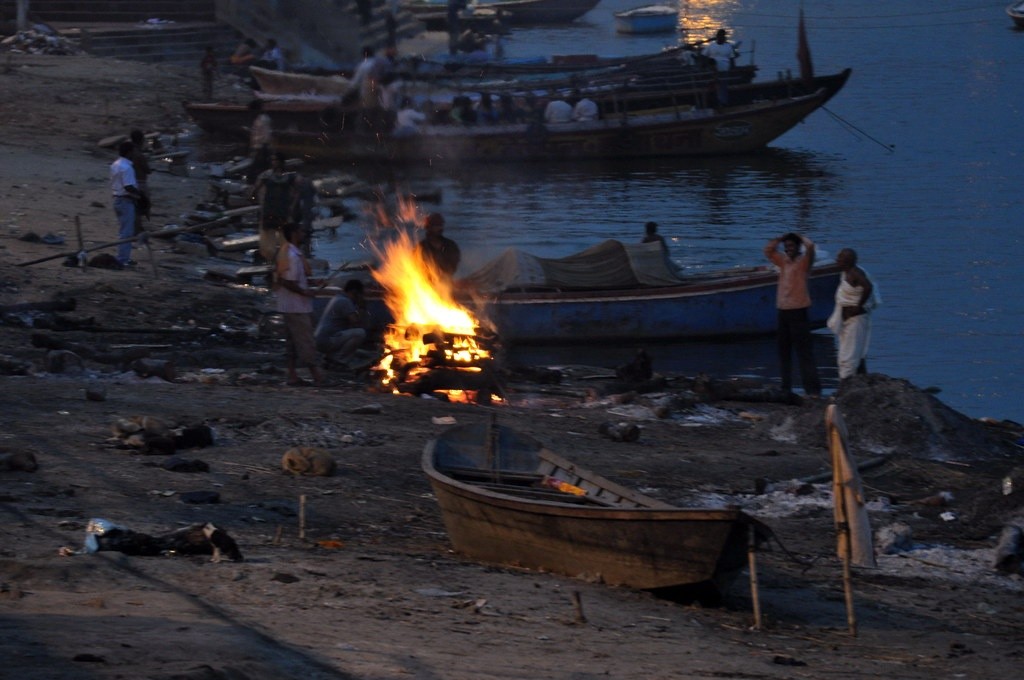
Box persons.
[231,38,285,90]
[274,224,327,384]
[244,103,274,182]
[200,47,217,97]
[343,44,598,130]
[677,28,737,73]
[109,141,140,265]
[131,130,154,234]
[249,158,316,265]
[417,212,460,276]
[641,221,668,249]
[827,247,880,388]
[314,279,367,359]
[765,231,822,399]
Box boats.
[615,5,679,34]
[314,264,859,342]
[1005,0,1024,30]
[420,422,771,590]
[408,0,601,22]
[184,44,853,165]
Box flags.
[795,19,813,80]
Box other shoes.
[127,258,137,265]
[810,391,820,399]
[284,377,310,386]
[121,260,133,269]
[322,354,335,362]
[311,377,340,387]
